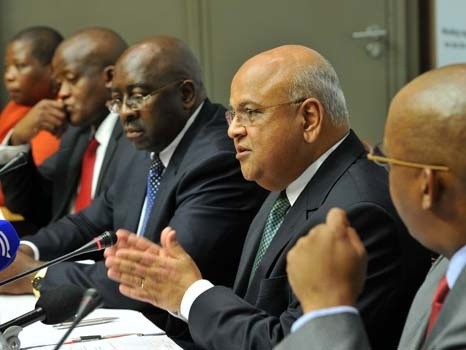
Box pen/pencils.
[53,315,119,331]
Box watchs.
[30,267,48,298]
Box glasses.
[366,145,450,172]
[224,98,307,128]
[105,79,184,114]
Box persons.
[0,24,466,350]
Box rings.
[141,279,145,288]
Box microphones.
[0,230,119,287]
[0,151,30,177]
[0,283,85,338]
[54,286,104,350]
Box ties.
[426,273,450,340]
[254,189,290,276]
[76,135,99,212]
[137,152,165,238]
